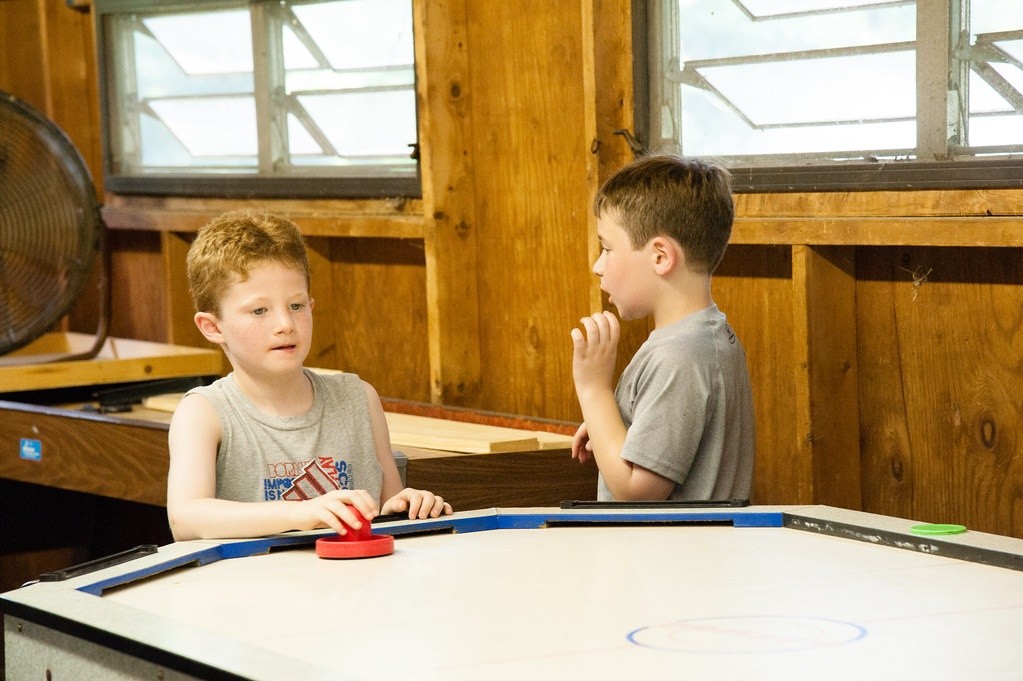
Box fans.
[0,90,111,367]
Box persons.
[166,210,453,543]
[571,153,753,506]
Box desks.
[0,328,598,521]
[0,501,1022,679]
